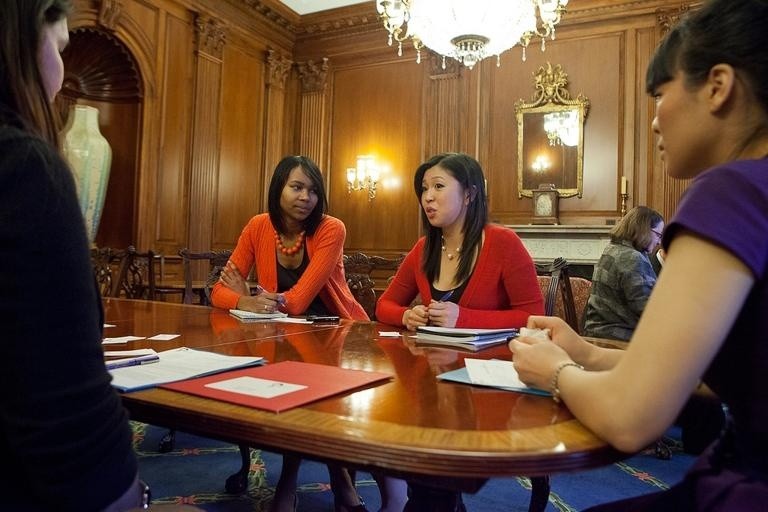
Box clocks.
[529,183,560,225]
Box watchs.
[139,479,153,510]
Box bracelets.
[549,360,584,407]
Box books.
[408,325,518,353]
[228,309,287,320]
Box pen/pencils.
[438,290,455,303]
[257,284,286,308]
[105,355,160,370]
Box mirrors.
[514,61,590,200]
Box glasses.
[650,228,663,238]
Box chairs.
[90,245,184,302]
[226,253,408,495]
[533,257,568,512]
[560,268,685,459]
[158,247,232,454]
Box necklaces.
[439,232,464,262]
[274,230,306,256]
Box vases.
[56,106,114,246]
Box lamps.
[376,0,568,70]
[346,155,400,202]
[544,111,578,148]
[531,155,552,176]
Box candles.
[484,179,487,197]
[621,176,626,194]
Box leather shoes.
[334,490,367,512]
[271,491,298,512]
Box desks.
[100,296,631,512]
[495,225,618,279]
[143,282,217,303]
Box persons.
[579,204,666,343]
[0,1,208,512]
[508,0,768,512]
[207,154,368,512]
[369,153,544,512]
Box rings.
[264,305,268,311]
